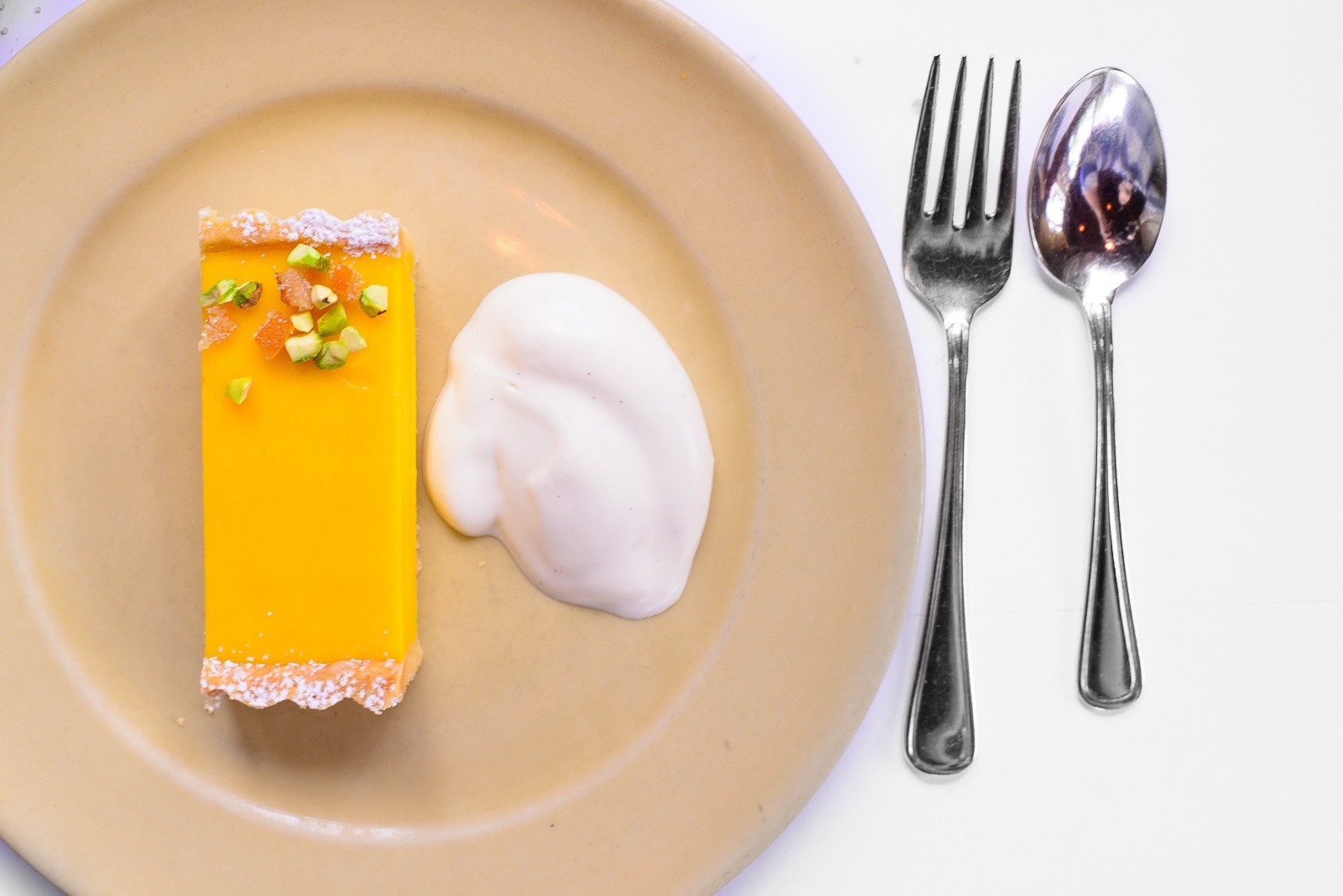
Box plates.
[0,1,929,896]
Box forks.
[901,53,1024,779]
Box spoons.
[1027,68,1169,714]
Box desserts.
[197,212,423,714]
